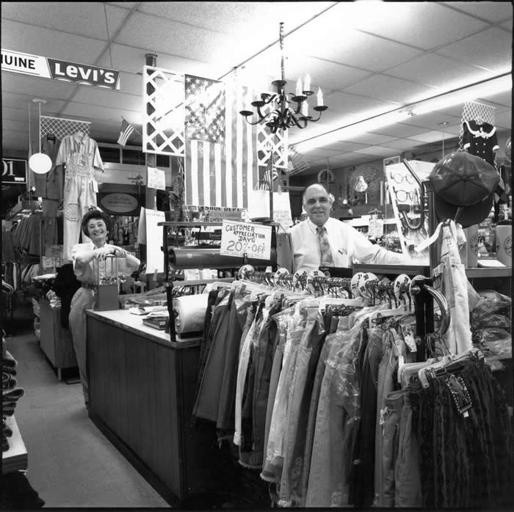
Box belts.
[81,281,98,289]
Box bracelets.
[111,249,117,254]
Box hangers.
[473,115,485,123]
[397,334,482,388]
[212,265,425,318]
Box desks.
[84,305,236,509]
[39,297,76,382]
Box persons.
[282,183,430,278]
[67,211,141,408]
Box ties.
[316,227,334,267]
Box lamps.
[29,98,52,174]
[238,22,328,134]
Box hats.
[431,152,500,228]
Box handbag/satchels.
[276,223,296,275]
